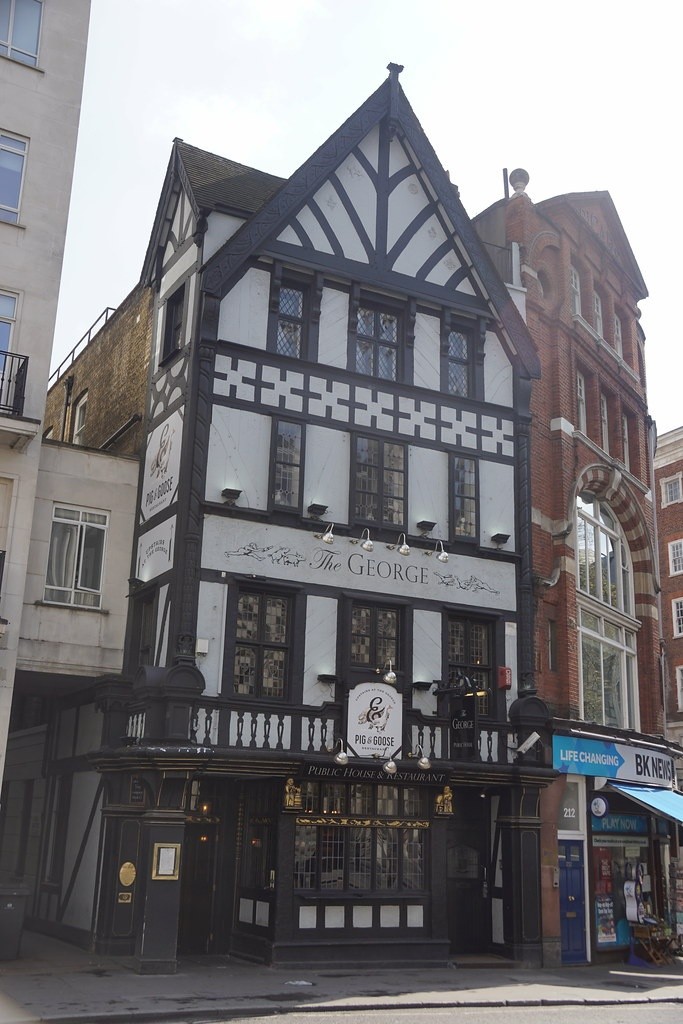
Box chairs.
[633,926,679,967]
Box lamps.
[314,522,334,544]
[376,659,397,685]
[374,747,397,775]
[327,738,348,765]
[416,520,436,532]
[425,540,449,563]
[317,674,338,684]
[408,745,431,769]
[386,533,410,556]
[307,504,328,515]
[220,488,243,500]
[491,534,511,544]
[348,527,374,553]
[412,681,433,692]
